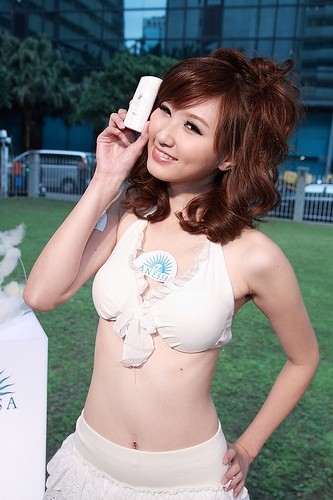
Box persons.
[22,47,320,500]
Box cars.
[276,170,333,218]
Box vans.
[7,149,97,195]
